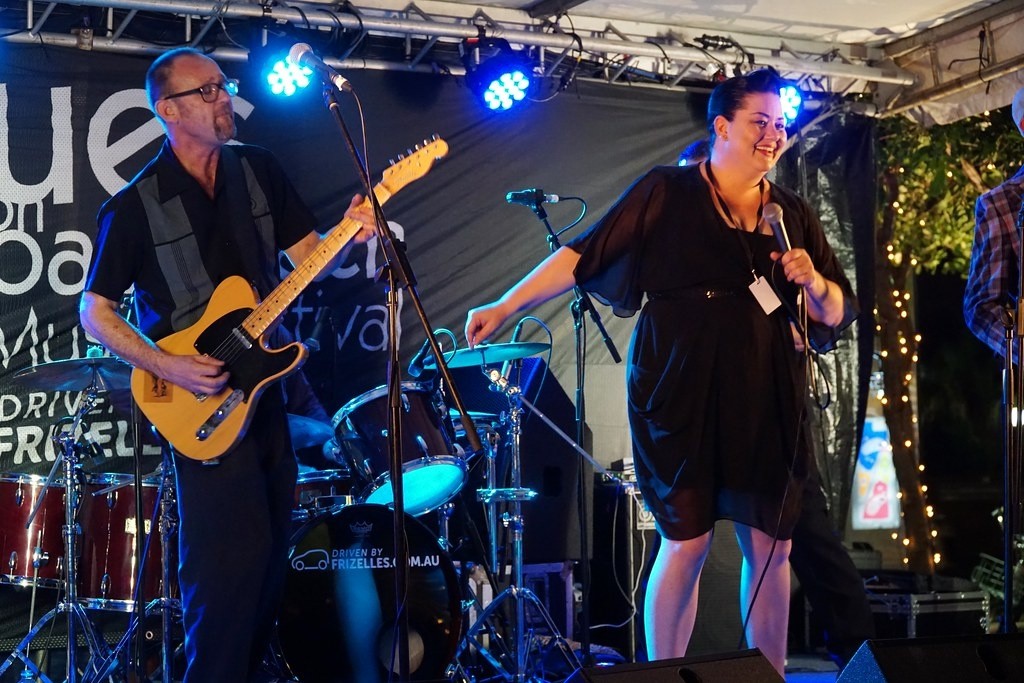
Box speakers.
[836,631,1024,683]
[592,482,748,662]
[566,648,786,683]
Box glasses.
[163,80,238,103]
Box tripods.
[433,350,594,683]
[0,369,191,683]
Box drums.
[294,470,358,516]
[264,503,463,683]
[1,471,69,592]
[72,471,184,620]
[333,376,470,519]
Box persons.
[640,139,873,666]
[80,47,380,683]
[963,87,1024,365]
[465,69,862,678]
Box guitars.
[130,132,450,466]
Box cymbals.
[285,411,333,451]
[10,356,134,394]
[425,341,551,371]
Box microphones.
[408,339,431,378]
[506,192,565,204]
[290,43,355,94]
[763,202,793,254]
[497,321,524,392]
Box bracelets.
[806,278,829,312]
